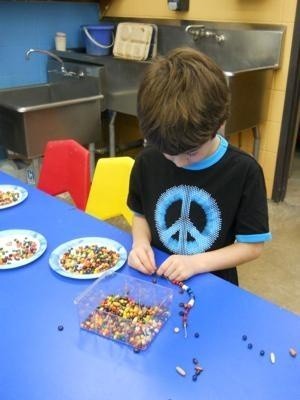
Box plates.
[0,184,128,280]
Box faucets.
[24,47,65,70]
[184,24,208,41]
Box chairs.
[86,156,135,224]
[39,139,90,212]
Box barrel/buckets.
[78,23,115,57]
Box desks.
[0,171,300,400]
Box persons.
[127,47,271,286]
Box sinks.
[0,82,103,160]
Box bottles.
[55,32,66,51]
